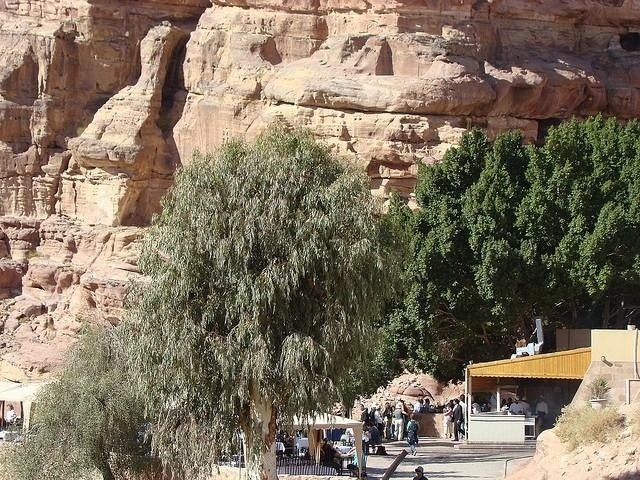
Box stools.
[350,468,366,477]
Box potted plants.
[583,377,612,410]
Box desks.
[275,441,285,452]
[339,454,355,477]
[296,438,309,456]
[340,427,372,453]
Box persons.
[276,390,466,479]
[4,402,18,431]
[470,390,561,438]
